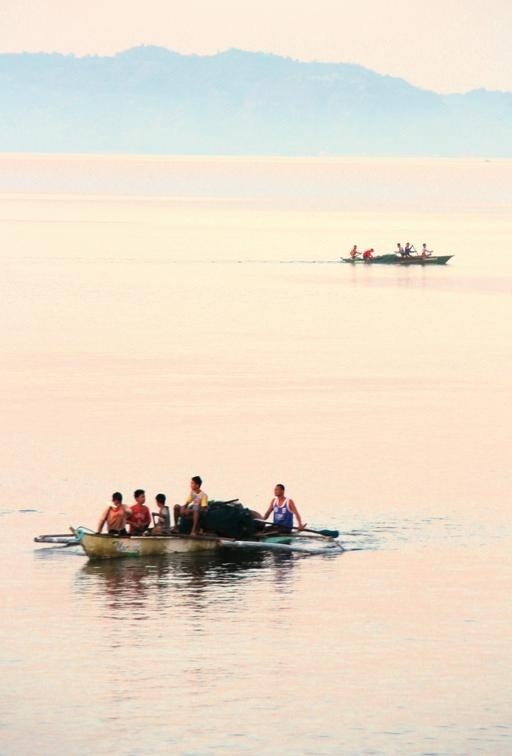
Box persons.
[144,494,170,536]
[394,243,404,258]
[96,492,132,535]
[262,484,304,535]
[351,245,361,260]
[422,244,433,257]
[170,476,208,536]
[125,490,151,536]
[363,248,374,264]
[405,243,416,258]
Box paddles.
[254,519,339,538]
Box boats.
[342,253,453,264]
[71,524,301,563]
